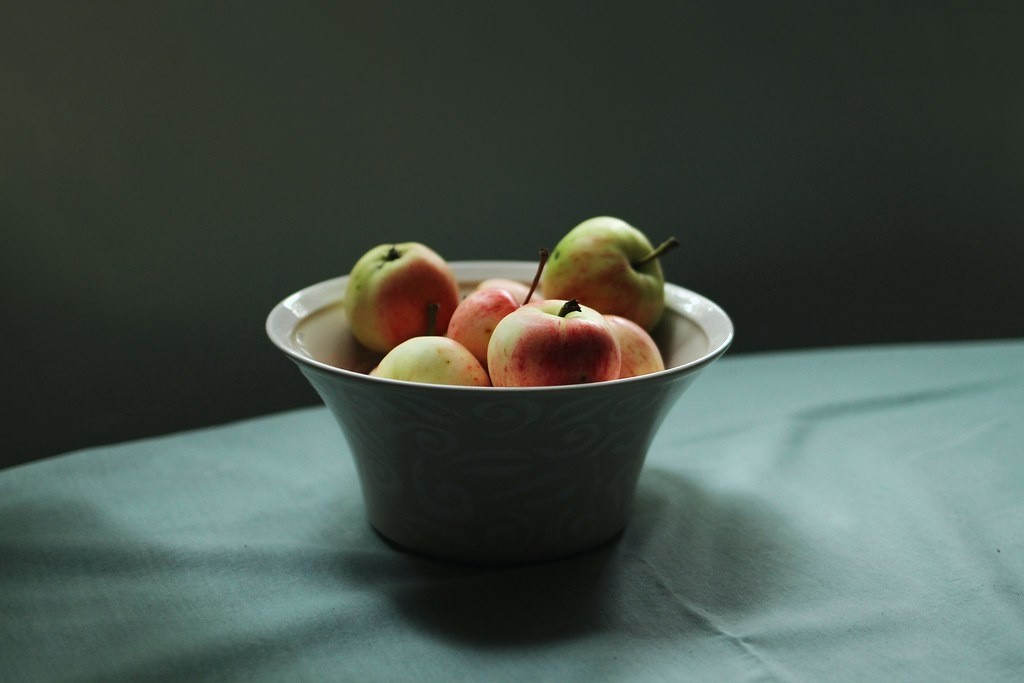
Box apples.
[346,215,681,388]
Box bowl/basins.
[265,261,735,551]
